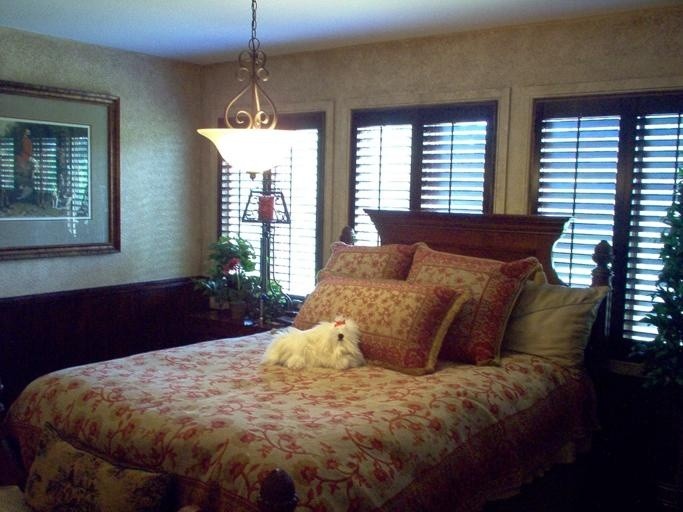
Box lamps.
[195,1,299,173]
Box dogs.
[261,315,366,371]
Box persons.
[13,127,38,203]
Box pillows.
[23,422,170,511]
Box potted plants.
[188,234,288,324]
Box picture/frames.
[0,77,122,258]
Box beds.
[12,210,615,512]
